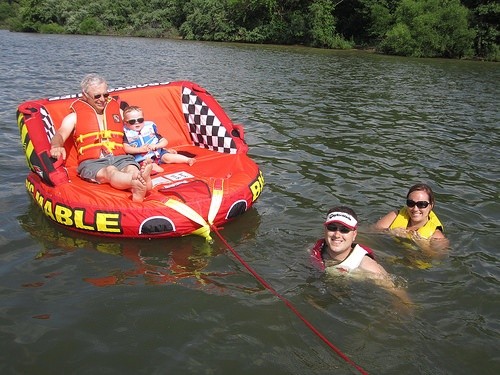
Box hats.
[323,211,358,231]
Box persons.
[50,73,153,202]
[308,206,411,306]
[376,184,449,258]
[123,106,196,175]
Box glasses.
[85,91,109,99]
[406,199,430,208]
[126,118,144,125]
[326,225,351,233]
